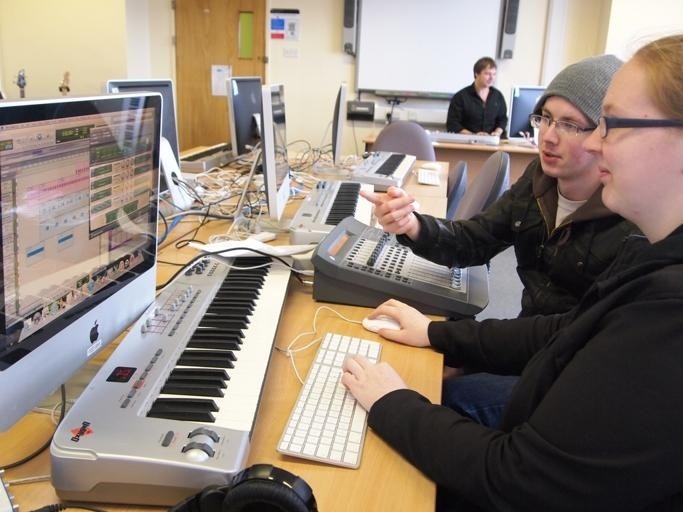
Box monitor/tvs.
[507,86,547,147]
[105,78,193,220]
[226,76,263,180]
[313,83,357,175]
[0,91,163,432]
[234,85,293,233]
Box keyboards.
[418,169,440,186]
[275,332,382,470]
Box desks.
[358,130,539,191]
[0,149,448,512]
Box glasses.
[596,114,680,137]
[529,113,595,136]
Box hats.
[534,50,624,124]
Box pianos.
[49,251,295,507]
[288,150,417,246]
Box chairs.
[447,159,468,217]
[369,120,437,161]
[446,150,510,221]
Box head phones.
[168,464,318,512]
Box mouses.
[423,162,440,170]
[363,314,402,334]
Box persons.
[360,54,625,429]
[342,33,682,511]
[446,57,508,138]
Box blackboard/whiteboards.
[355,0,505,99]
